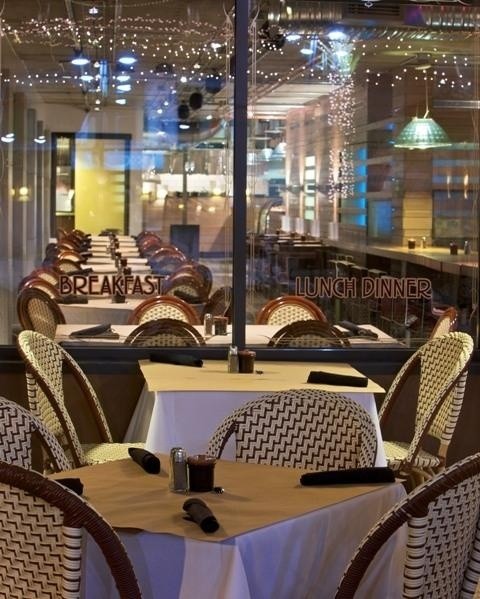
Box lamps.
[391,63,453,149]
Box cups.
[408,238,416,249]
[187,454,217,492]
[110,238,132,276]
[239,350,256,373]
[213,316,228,335]
[450,243,458,255]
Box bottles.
[170,446,188,492]
[464,241,472,255]
[228,345,239,374]
[421,236,427,248]
[205,313,212,336]
